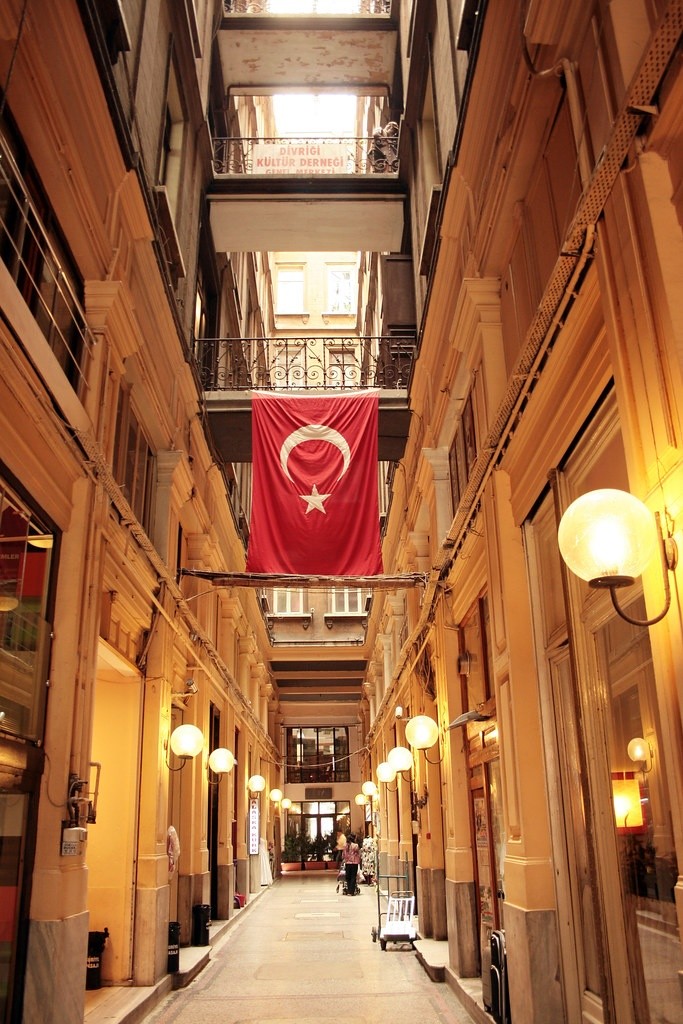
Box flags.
[247,388,384,578]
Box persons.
[334,829,347,872]
[341,833,360,895]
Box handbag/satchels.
[355,867,365,883]
[336,869,345,881]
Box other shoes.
[346,891,354,896]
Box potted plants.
[279,832,303,871]
[327,832,343,869]
[303,835,327,870]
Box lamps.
[556,487,680,631]
[396,706,411,722]
[164,725,204,771]
[388,747,416,784]
[269,789,283,808]
[277,798,291,811]
[355,794,369,810]
[375,762,400,791]
[205,747,233,784]
[627,737,655,774]
[403,715,446,766]
[362,780,379,803]
[246,775,266,799]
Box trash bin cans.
[191,904,211,946]
[83,927,109,989]
[167,921,180,973]
